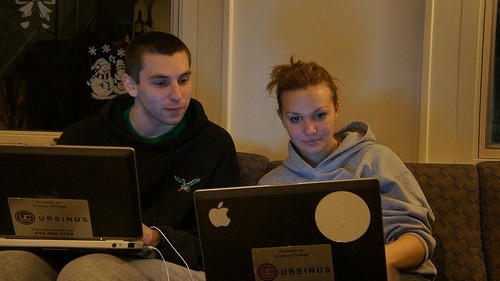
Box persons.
[1,32,242,281]
[255,53,437,281]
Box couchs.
[236,152,500,281]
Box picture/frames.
[0,0,184,148]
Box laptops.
[0,143,147,251]
[193,179,389,281]
